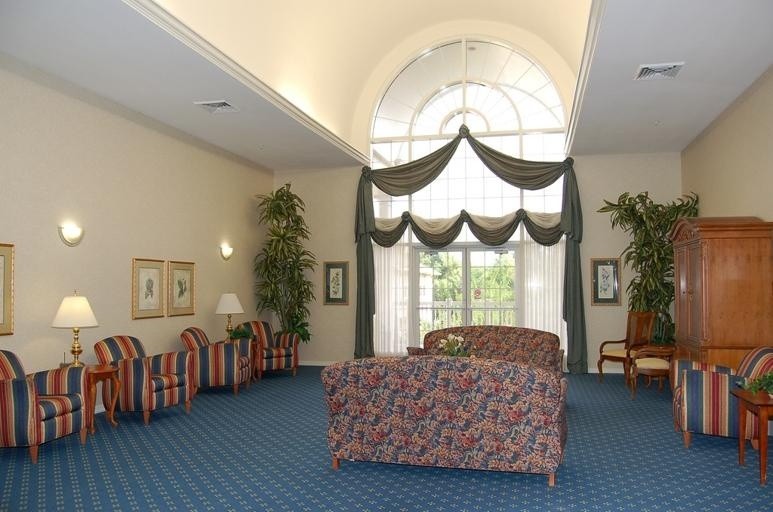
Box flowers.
[437,334,472,356]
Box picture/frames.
[132,257,166,320]
[323,261,349,305]
[167,261,195,317]
[591,258,621,306]
[0,243,15,336]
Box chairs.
[630,344,676,401]
[0,350,87,464]
[180,327,253,397]
[598,310,656,389]
[236,320,300,382]
[94,336,194,426]
[670,345,773,451]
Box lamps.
[216,293,245,341]
[51,289,99,368]
[57,226,85,247]
[220,247,233,261]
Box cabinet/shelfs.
[667,216,773,392]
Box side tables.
[60,363,121,435]
[219,341,257,385]
[730,389,773,487]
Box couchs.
[320,356,569,487]
[406,326,564,374]
